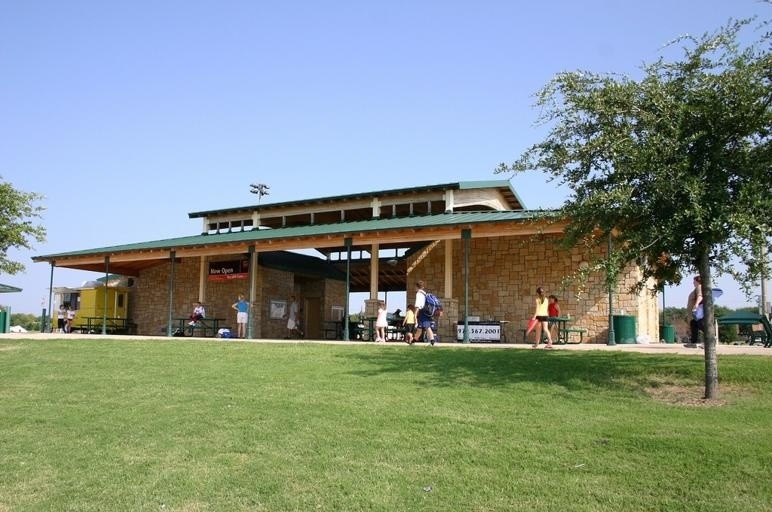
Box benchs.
[384,328,407,342]
[321,329,337,339]
[76,323,128,334]
[353,329,366,339]
[519,328,546,344]
[559,329,588,344]
[171,325,233,337]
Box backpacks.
[421,291,444,318]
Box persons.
[392,281,435,345]
[283,296,304,340]
[375,300,388,343]
[683,276,705,349]
[544,295,564,343]
[66,306,75,333]
[231,293,253,339]
[531,287,553,348]
[57,305,66,333]
[188,302,206,326]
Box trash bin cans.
[659,326,675,343]
[613,315,636,344]
[0,312,6,333]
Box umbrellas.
[525,316,538,336]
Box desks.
[360,318,392,341]
[80,316,133,334]
[536,316,575,344]
[173,317,226,337]
[323,319,358,340]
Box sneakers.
[375,337,385,342]
[684,342,698,348]
[409,340,416,345]
[430,339,435,345]
[188,321,197,326]
[237,336,246,338]
[532,344,538,347]
[283,332,304,340]
[545,345,552,348]
